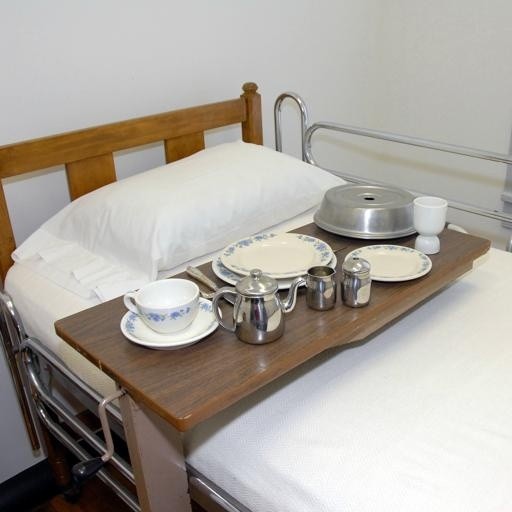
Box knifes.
[185,266,237,307]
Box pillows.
[9,138,347,303]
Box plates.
[119,296,218,351]
[211,232,338,289]
[344,244,433,284]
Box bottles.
[343,258,372,308]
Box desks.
[53,224,491,512]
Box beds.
[0,80,512,512]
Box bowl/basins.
[313,182,420,240]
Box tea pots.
[211,267,306,347]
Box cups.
[306,264,339,312]
[413,195,447,254]
[122,277,200,336]
